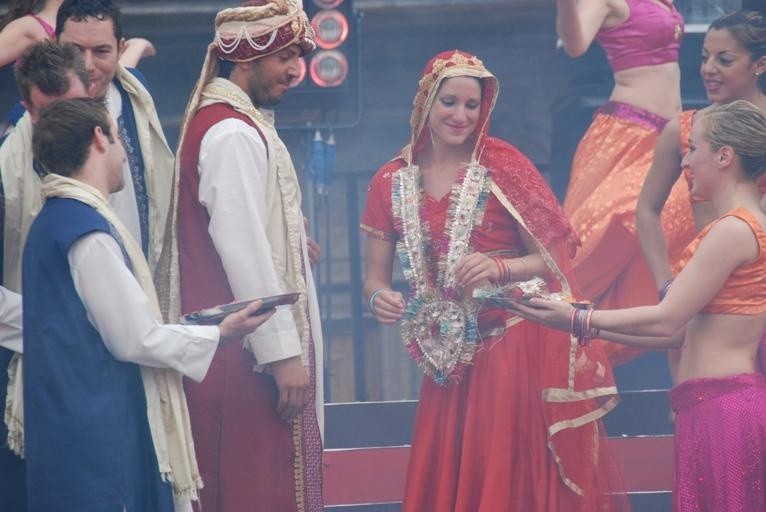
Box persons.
[545,2,686,362]
[176,1,330,512]
[505,100,764,512]
[1,1,280,512]
[633,12,766,358]
[360,47,621,509]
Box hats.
[214,0,316,62]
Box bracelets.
[366,288,386,314]
[570,306,592,336]
[657,278,674,298]
[491,256,514,284]
[573,323,601,346]
